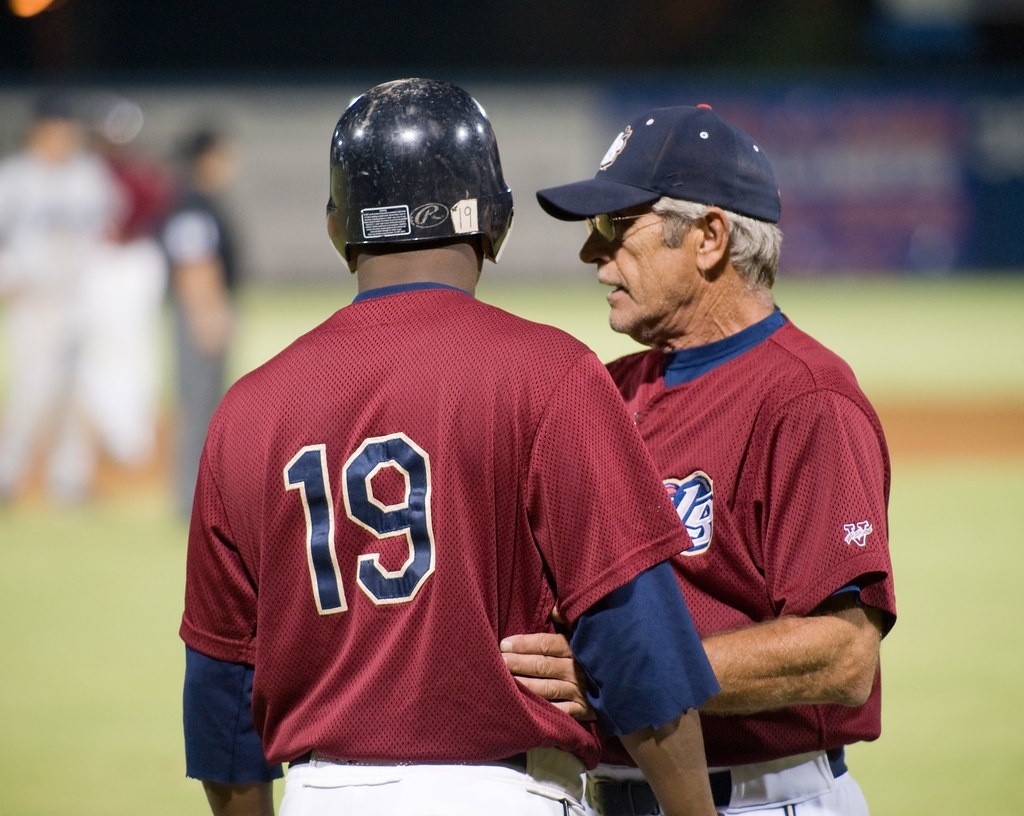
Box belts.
[590,745,849,816]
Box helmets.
[325,77,514,272]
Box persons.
[500,103,899,816]
[0,99,242,524]
[179,78,722,816]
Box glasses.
[586,210,675,241]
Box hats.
[537,105,782,226]
